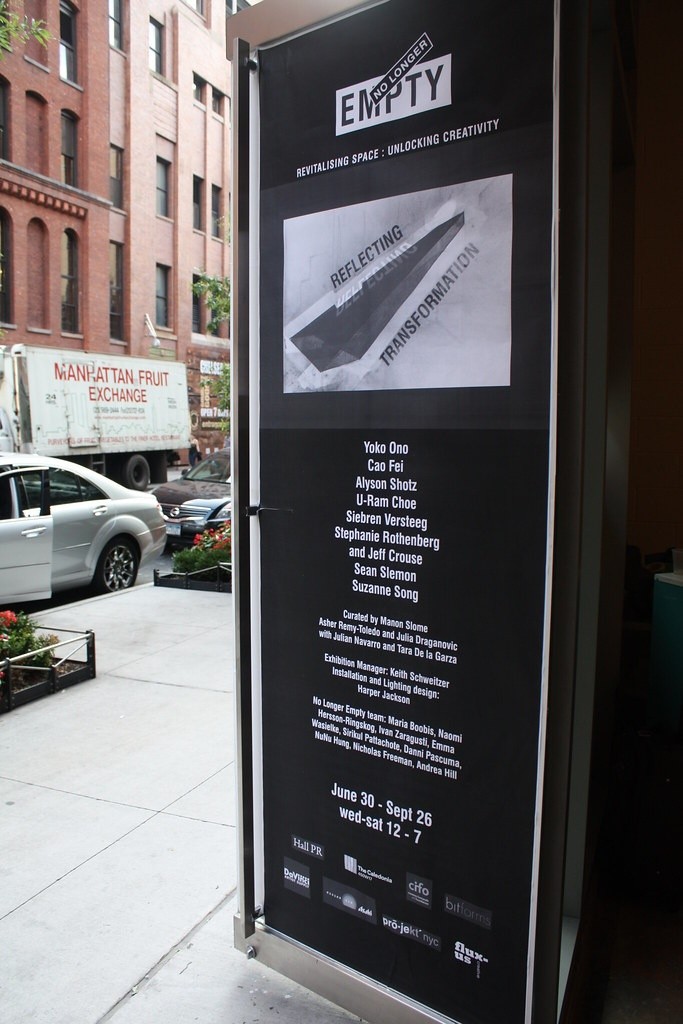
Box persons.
[173,451,181,471]
[187,430,230,468]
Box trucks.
[1,342,193,492]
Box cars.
[0,452,168,607]
[149,446,231,550]
